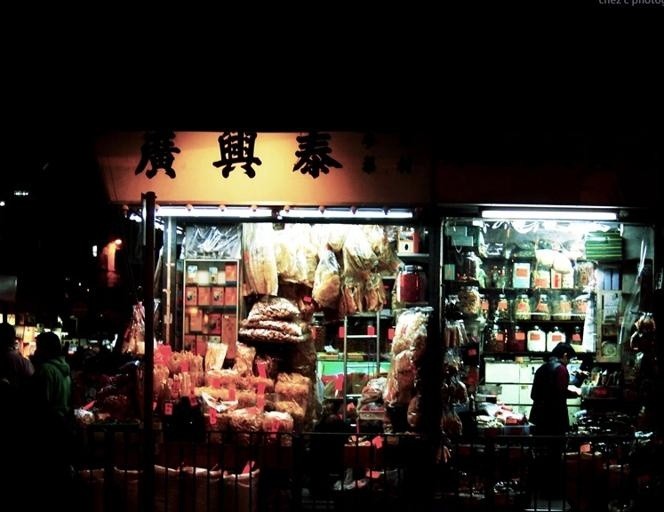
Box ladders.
[343,312,380,424]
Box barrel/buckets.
[525,326,546,352]
[546,325,567,353]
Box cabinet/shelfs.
[443,232,654,416]
[182,255,242,365]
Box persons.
[0,322,71,420]
[529,343,578,432]
